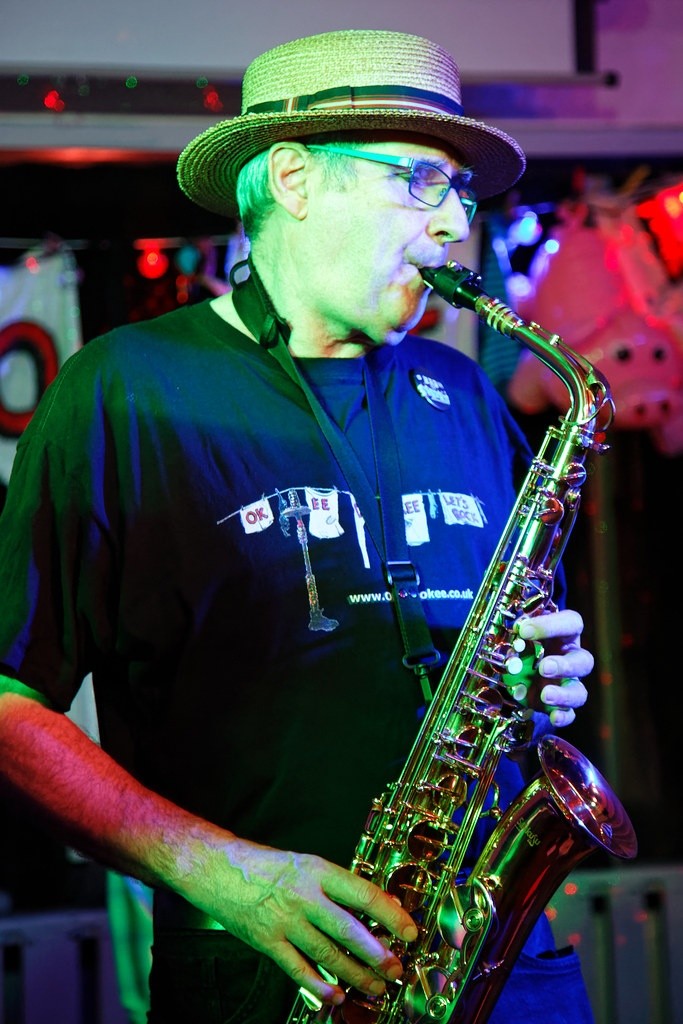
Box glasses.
[306,144,477,226]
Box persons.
[0,29,597,1024]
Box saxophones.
[284,257,640,1024]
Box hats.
[176,30,526,221]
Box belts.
[153,875,511,931]
